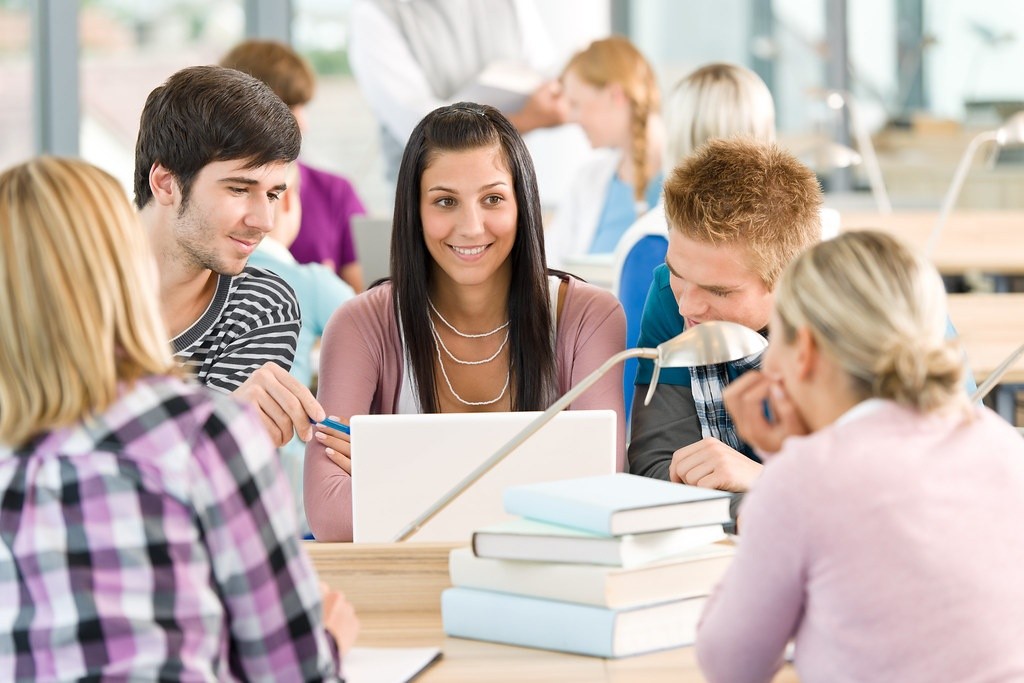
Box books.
[448,548,740,607]
[442,587,717,658]
[472,519,727,568]
[502,472,733,538]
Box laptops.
[351,410,617,545]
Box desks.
[301,543,798,683]
[861,205,1023,426]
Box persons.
[610,62,840,425]
[302,101,627,541]
[246,158,356,385]
[694,230,1024,683]
[1,158,363,683]
[560,36,665,291]
[345,0,633,215]
[625,141,823,529]
[220,42,371,295]
[130,65,326,447]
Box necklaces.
[426,290,513,405]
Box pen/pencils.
[310,415,350,435]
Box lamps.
[389,323,770,540]
[924,109,1023,261]
